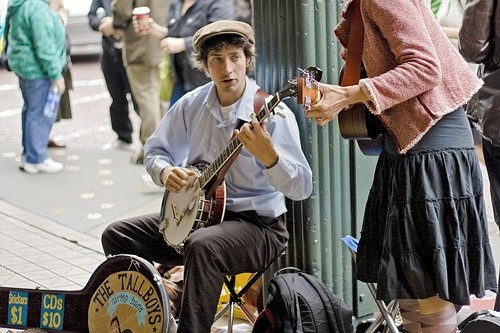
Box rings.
[318,116,324,121]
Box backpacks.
[252,267,354,333]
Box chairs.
[338,235,401,333]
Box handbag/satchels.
[458,310,500,333]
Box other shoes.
[130,150,145,164]
[19,156,26,168]
[24,157,63,173]
[48,139,65,147]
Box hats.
[192,20,255,55]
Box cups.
[132,6,151,33]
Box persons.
[88,0,169,163]
[102,20,316,333]
[4,0,73,175]
[132,0,238,110]
[305,0,500,333]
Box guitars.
[296,62,384,157]
[158,65,322,255]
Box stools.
[213,250,287,333]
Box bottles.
[43,83,61,119]
